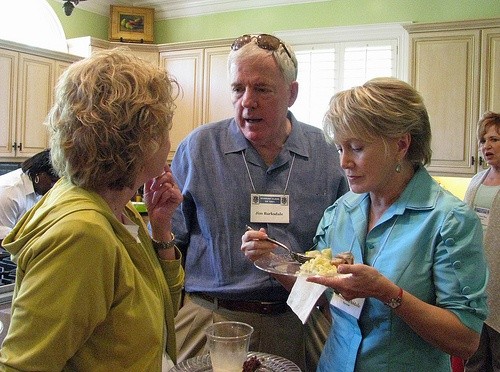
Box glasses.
[231,34,296,67]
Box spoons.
[245,224,315,263]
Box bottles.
[135,191,141,202]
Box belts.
[190,292,292,315]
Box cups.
[205,321,254,372]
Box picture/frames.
[109,5,154,43]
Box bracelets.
[152,233,175,249]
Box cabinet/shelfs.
[401,16,500,179]
[0,35,238,180]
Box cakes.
[297,248,338,276]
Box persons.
[0,148,61,242]
[0,46,186,372]
[463,110,499,372]
[146,34,349,372]
[241,76,490,372]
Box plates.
[168,351,301,372]
[254,254,321,277]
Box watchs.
[386,289,404,309]
